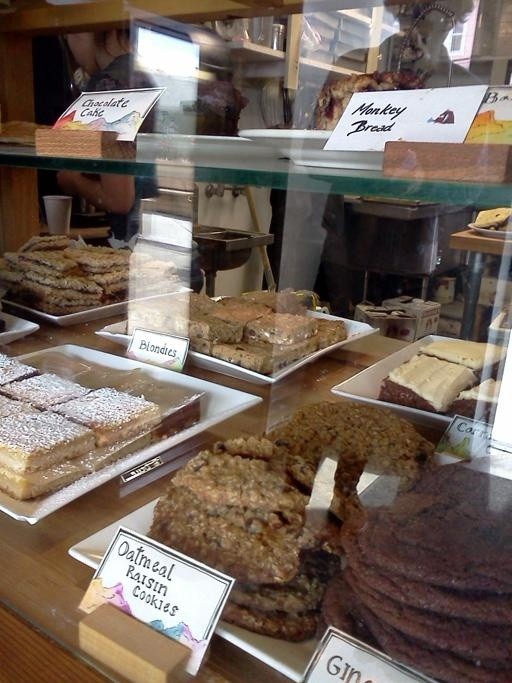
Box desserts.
[376,338,508,425]
[0,235,180,316]
[145,399,512,682]
[0,351,205,502]
[124,292,346,376]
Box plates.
[215,18,247,41]
[135,126,384,173]
[95,295,381,384]
[1,284,195,326]
[1,343,265,524]
[469,222,510,237]
[330,333,504,433]
[67,449,462,683]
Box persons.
[51,23,204,296]
[281,0,479,531]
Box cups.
[249,15,273,47]
[432,276,458,303]
[43,194,75,236]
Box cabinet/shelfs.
[0,0,512,683]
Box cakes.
[314,69,422,131]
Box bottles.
[273,23,286,52]
